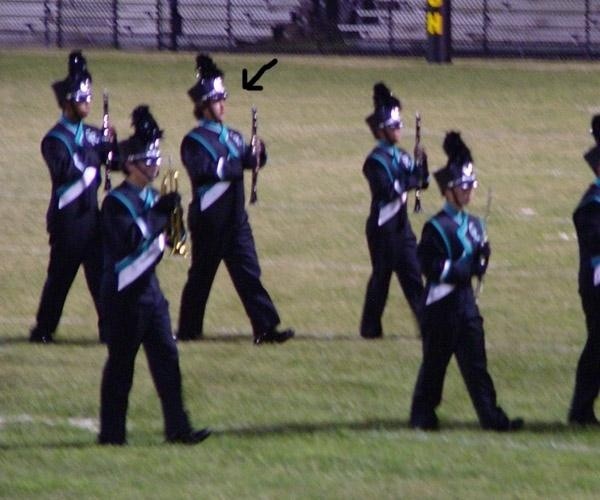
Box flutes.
[102,88,112,193]
[470,187,492,306]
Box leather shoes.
[505,418,524,432]
[253,327,295,345]
[567,416,600,428]
[30,328,56,344]
[166,428,211,444]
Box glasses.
[388,122,403,129]
[145,158,162,167]
[461,181,478,191]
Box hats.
[187,53,227,108]
[583,115,599,170]
[116,106,162,162]
[434,131,478,190]
[365,83,403,131]
[51,50,92,100]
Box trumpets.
[162,155,190,257]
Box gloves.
[154,193,176,214]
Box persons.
[566,112,599,429]
[176,52,297,347]
[359,79,432,338]
[26,48,121,347]
[92,96,214,449]
[407,127,529,434]
